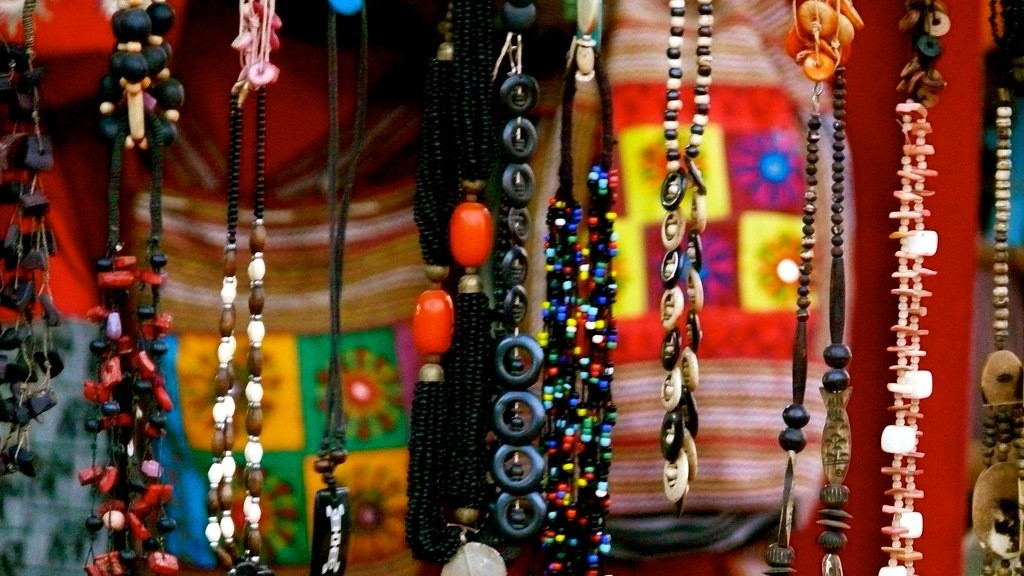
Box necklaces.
[84,0,178,576]
[0,2,67,481]
[206,0,621,576]
[874,1,949,576]
[658,0,712,516]
[970,1,1024,576]
[763,0,865,576]
